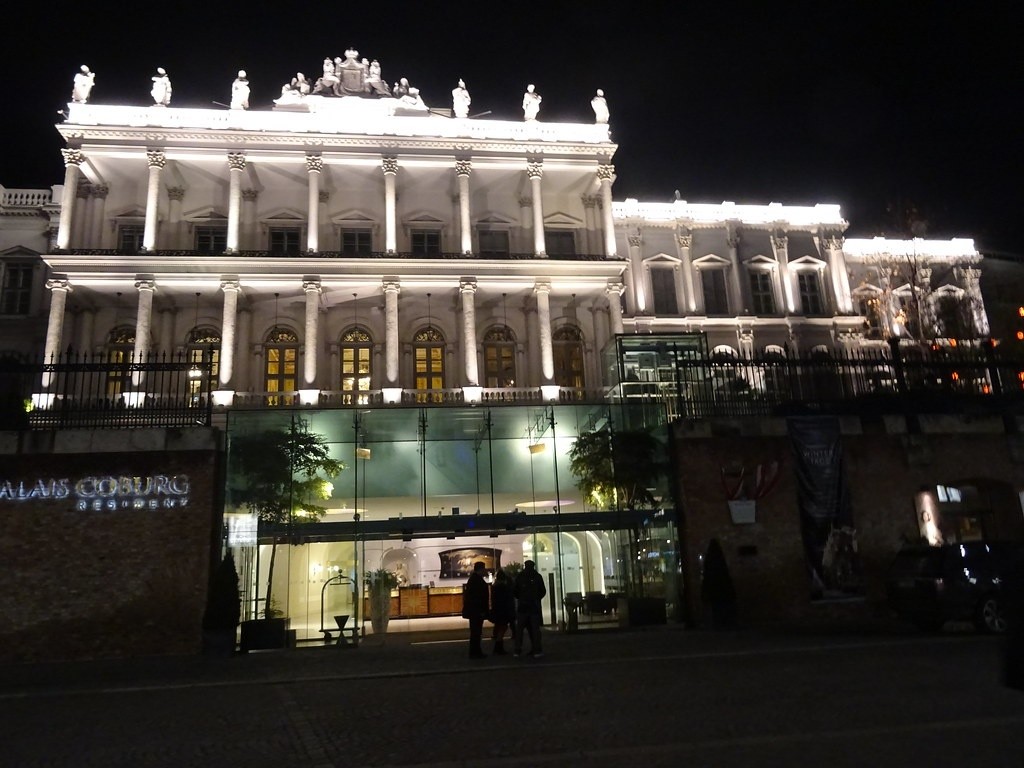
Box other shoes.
[469,651,488,660]
[512,651,544,659]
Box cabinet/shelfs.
[363,584,463,619]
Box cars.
[879,533,1017,639]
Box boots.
[493,640,507,655]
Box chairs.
[567,591,584,614]
[607,592,618,612]
[586,591,605,615]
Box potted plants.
[366,566,399,633]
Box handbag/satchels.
[517,604,527,613]
[488,609,501,624]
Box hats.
[526,561,536,567]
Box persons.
[514,560,546,657]
[591,89,610,124]
[487,568,516,657]
[72,65,96,104]
[523,84,541,120]
[282,57,410,100]
[461,561,490,660]
[230,70,250,110]
[151,68,172,107]
[452,78,471,117]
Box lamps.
[528,426,545,453]
[355,433,371,460]
